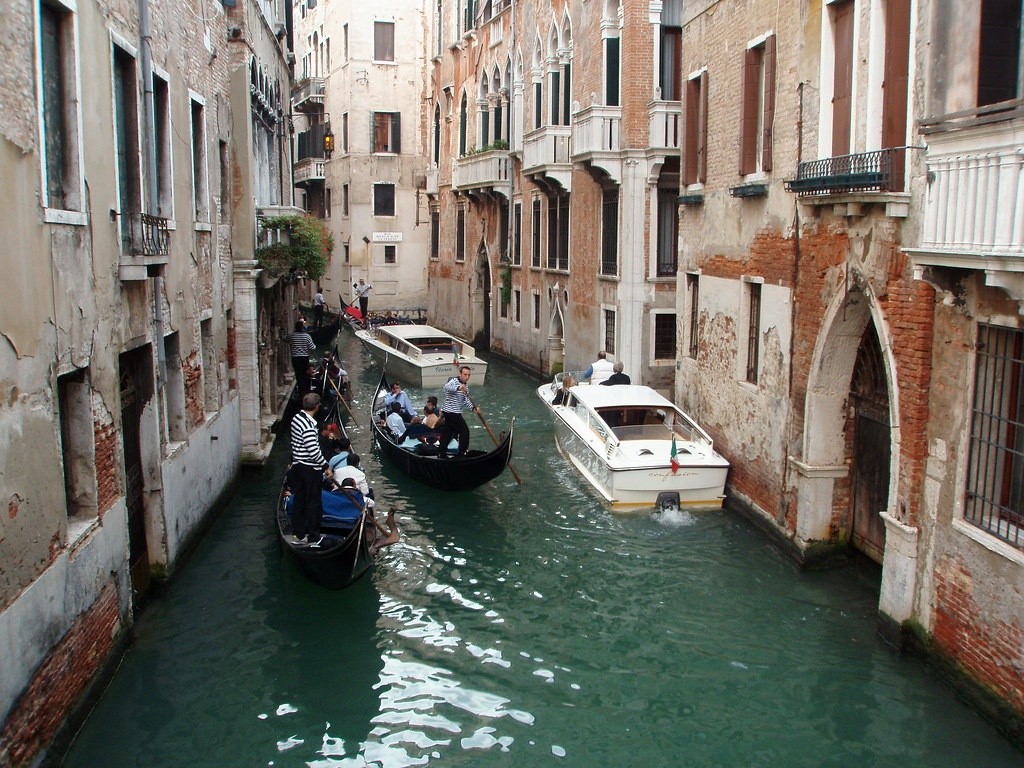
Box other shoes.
[308,542,318,545]
[291,535,307,544]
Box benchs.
[283,484,365,523]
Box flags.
[454,347,459,367]
[670,437,680,472]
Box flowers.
[255,211,334,281]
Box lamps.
[283,113,335,160]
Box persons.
[286,321,315,399]
[437,366,480,459]
[385,402,405,438]
[553,377,577,406]
[312,287,325,328]
[310,350,348,396]
[415,433,439,456]
[403,396,444,438]
[355,278,372,318]
[599,362,631,385]
[579,351,615,385]
[382,382,417,422]
[283,393,374,547]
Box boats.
[283,337,351,446]
[535,371,732,516]
[369,351,516,490]
[355,326,489,392]
[298,299,429,347]
[275,375,402,589]
